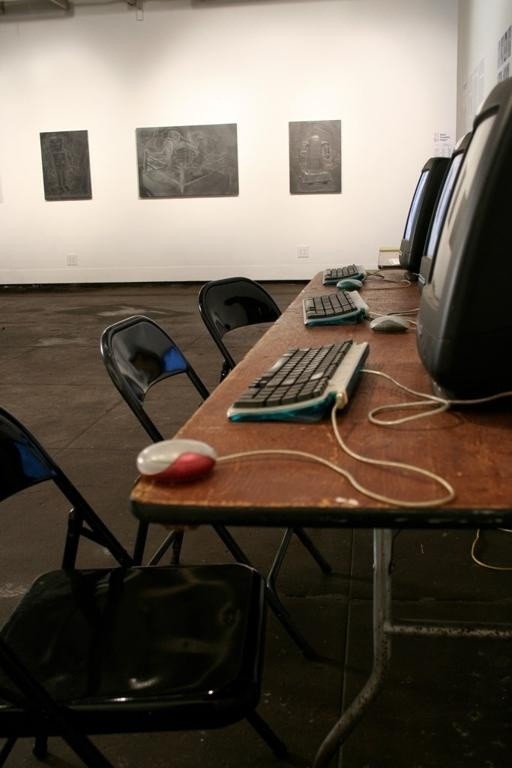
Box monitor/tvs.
[415,76,511,414]
[398,156,451,283]
[416,131,473,295]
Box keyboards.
[322,264,367,286]
[302,289,370,327]
[226,339,370,424]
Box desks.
[128,267,512,767]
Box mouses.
[336,279,363,291]
[136,438,217,484]
[370,314,409,332]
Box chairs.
[199,276,282,389]
[1,408,298,766]
[100,315,337,663]
